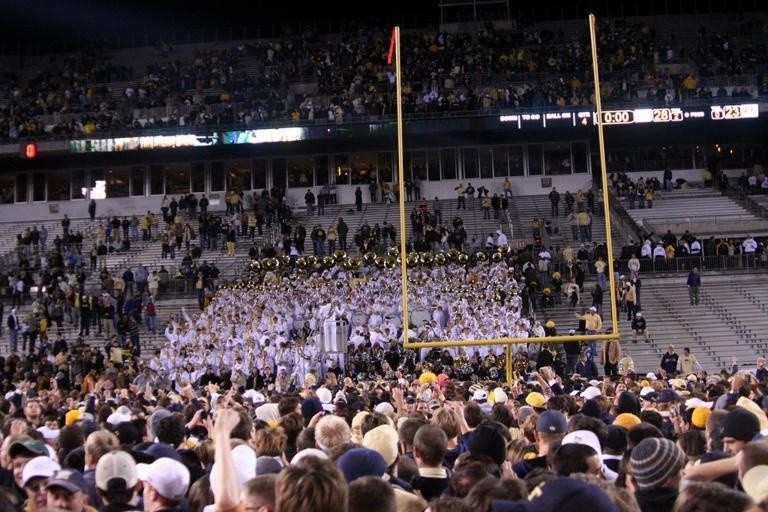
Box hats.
[628,436,691,493]
[635,312,642,316]
[471,389,488,403]
[8,440,189,503]
[488,472,624,512]
[589,306,597,312]
[681,397,714,415]
[717,407,761,444]
[569,330,575,334]
[535,408,571,435]
[362,424,400,468]
[580,386,602,401]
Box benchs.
[1,180,766,381]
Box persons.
[1,1,768,510]
